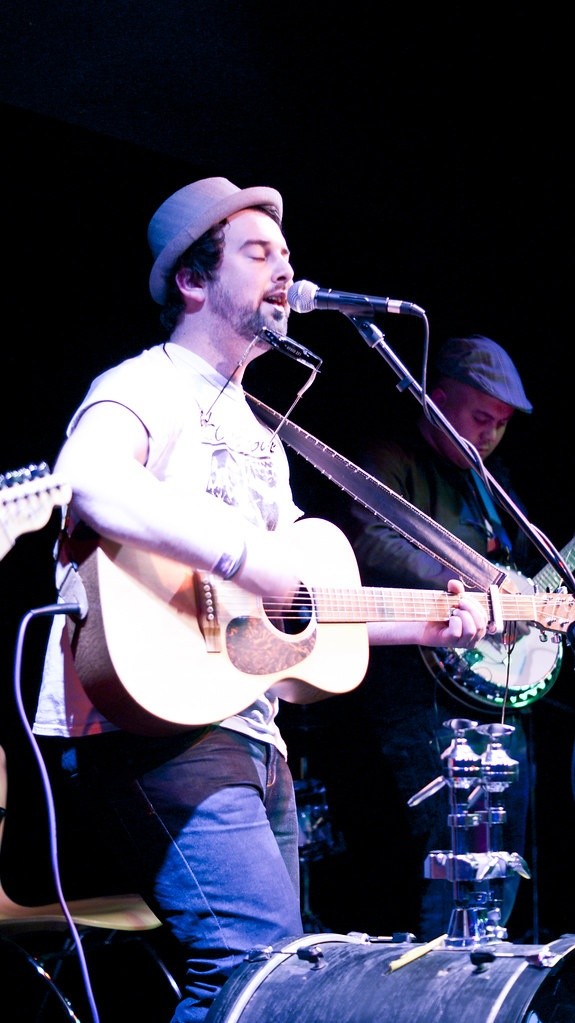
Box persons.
[27,176,489,1023]
[347,337,533,937]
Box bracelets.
[209,539,246,581]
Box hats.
[147,176,284,306]
[434,333,534,415]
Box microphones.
[286,280,425,320]
